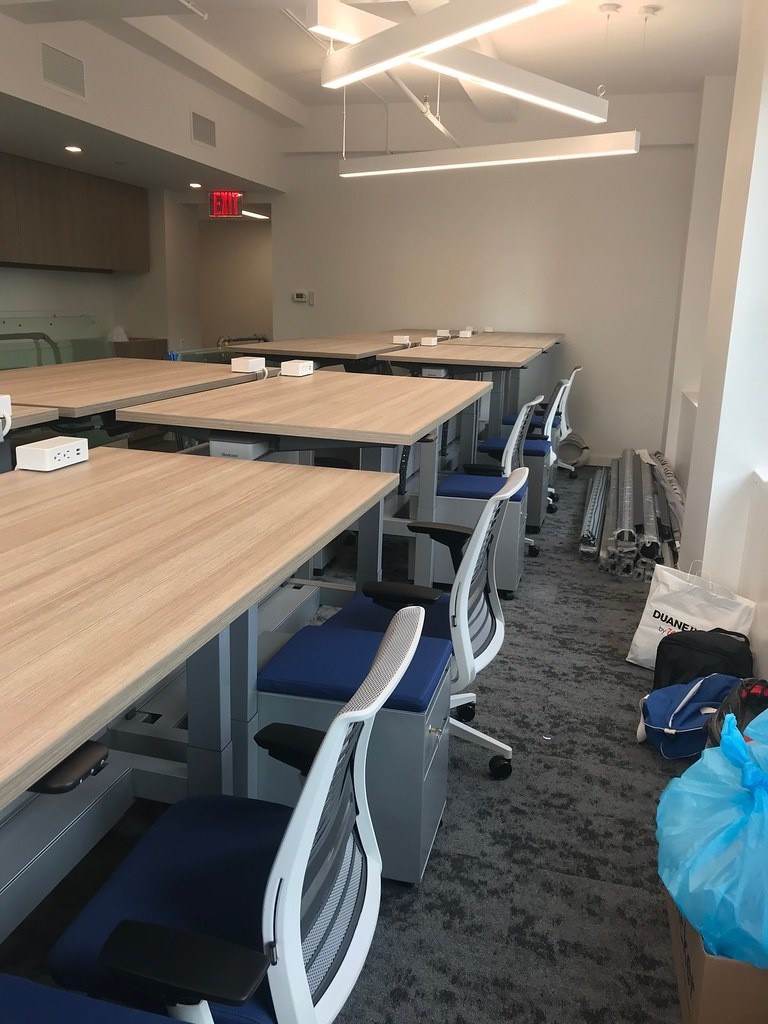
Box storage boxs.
[666,884,768,1024]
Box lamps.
[304,1,661,179]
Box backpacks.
[637,628,768,759]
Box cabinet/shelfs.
[407,474,529,601]
[500,414,562,485]
[256,624,453,887]
[479,436,552,534]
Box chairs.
[407,394,545,556]
[320,467,530,779]
[40,606,425,1024]
[476,366,584,514]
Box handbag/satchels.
[626,560,756,671]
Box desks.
[116,371,493,590]
[0,357,281,456]
[225,328,478,475]
[375,343,543,474]
[0,446,400,949]
[0,405,60,476]
[436,331,564,421]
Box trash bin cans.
[207,434,271,461]
